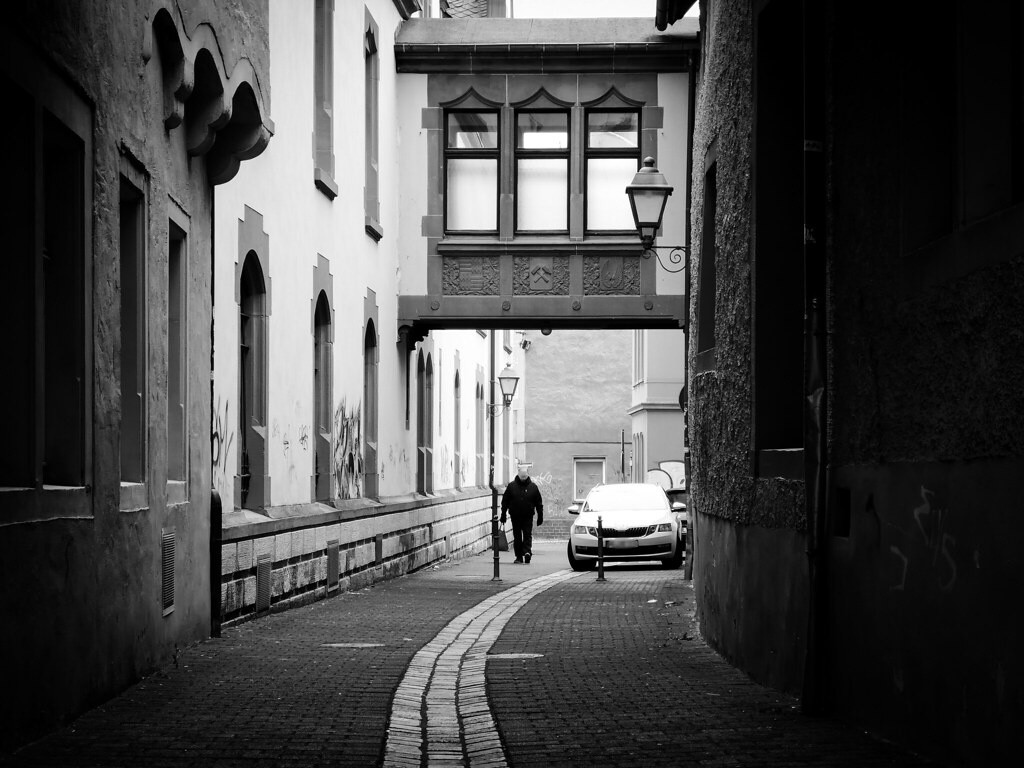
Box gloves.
[500,515,507,523]
[537,517,543,526]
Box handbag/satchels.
[492,523,509,551]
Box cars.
[567,482,687,570]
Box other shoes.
[515,555,523,563]
[525,555,530,563]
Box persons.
[500,468,544,564]
